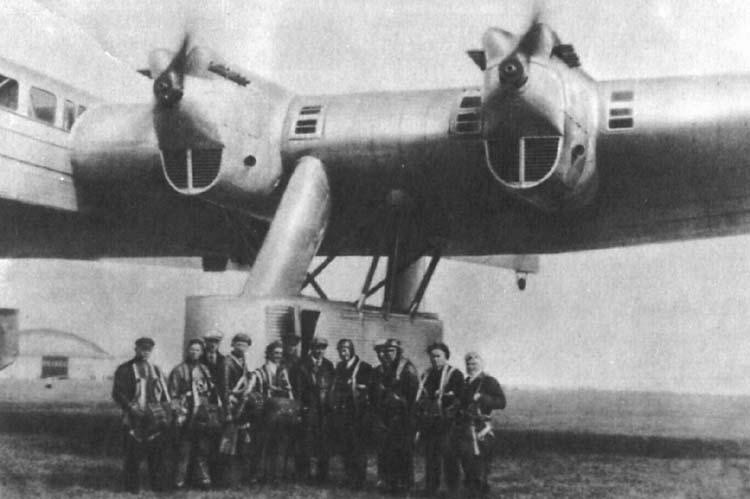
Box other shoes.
[334,474,489,497]
[124,472,234,494]
[237,471,332,488]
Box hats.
[374,336,404,352]
[135,338,155,347]
[427,342,450,360]
[187,331,355,362]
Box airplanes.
[0,2,750,457]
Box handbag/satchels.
[261,398,298,440]
[241,393,265,422]
[191,401,224,435]
[143,399,178,439]
